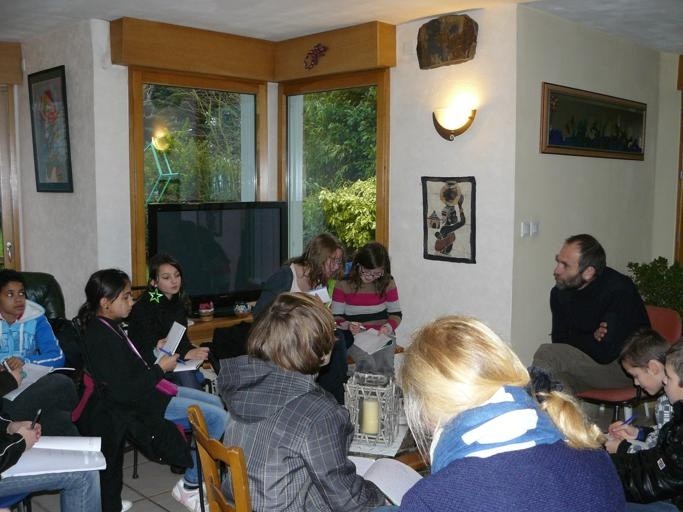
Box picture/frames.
[539,80,648,160]
[23,65,76,192]
[422,174,477,264]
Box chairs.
[184,406,248,512]
[577,304,683,443]
[0,495,33,512]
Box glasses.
[328,256,342,265]
[360,264,384,278]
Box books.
[154,321,186,364]
[49,367,76,373]
[306,285,331,303]
[354,328,394,355]
[1,436,107,479]
[3,363,54,402]
[173,359,204,372]
[347,456,423,507]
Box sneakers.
[171,479,209,512]
[121,499,132,512]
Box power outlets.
[521,223,530,236]
[530,223,538,236]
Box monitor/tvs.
[148,201,288,319]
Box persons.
[75,269,229,512]
[218,292,386,512]
[530,233,653,392]
[128,257,211,390]
[0,357,80,436]
[253,232,353,404]
[330,242,403,378]
[389,314,628,512]
[0,268,65,369]
[0,412,103,512]
[600,339,683,512]
[607,328,675,454]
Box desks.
[134,298,271,350]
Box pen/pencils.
[349,321,368,331]
[621,414,638,425]
[158,347,185,364]
[30,408,41,430]
[2,360,13,374]
[189,342,200,349]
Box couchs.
[1,270,81,384]
